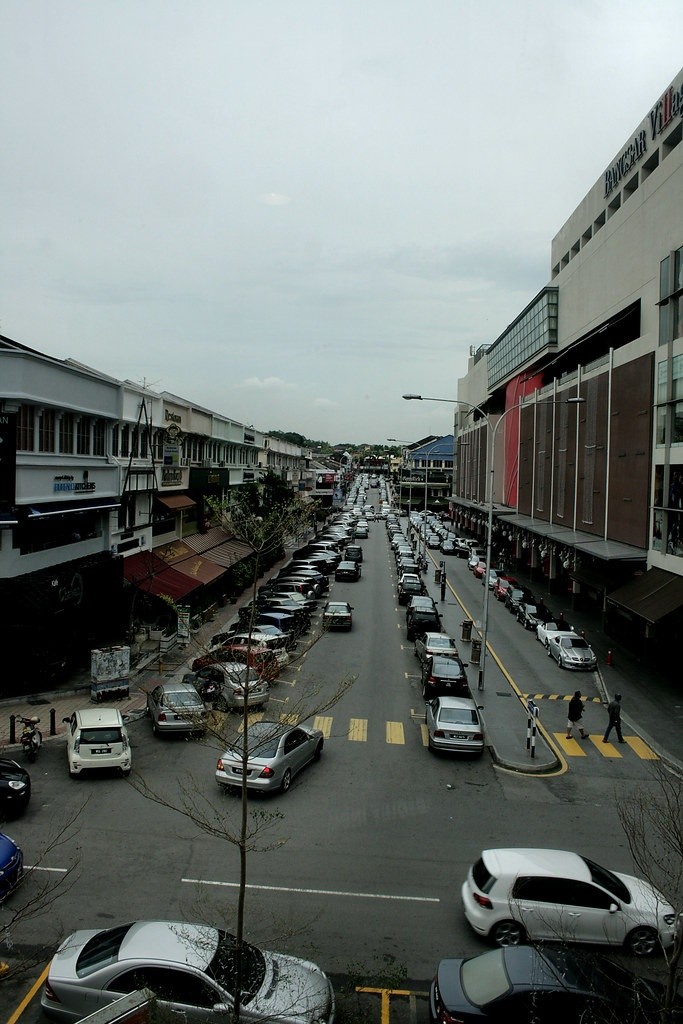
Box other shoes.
[566,735,573,739]
[618,740,627,744]
[602,739,609,743]
[581,734,589,740]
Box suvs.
[62,708,132,779]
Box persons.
[373,515,380,523]
[601,692,627,744]
[565,691,589,739]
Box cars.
[215,721,325,797]
[424,696,485,761]
[370,474,443,643]
[335,561,362,582]
[147,683,208,738]
[0,832,24,898]
[208,473,374,672]
[409,509,597,672]
[344,546,363,562]
[0,758,31,813]
[461,847,679,959]
[40,920,336,1024]
[419,655,470,699]
[321,601,355,632]
[414,632,459,661]
[191,644,280,683]
[182,662,270,713]
[430,944,683,1024]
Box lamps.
[459,510,574,569]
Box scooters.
[13,715,43,764]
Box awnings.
[605,567,683,639]
[158,494,197,508]
[123,525,257,604]
[497,513,647,561]
[445,496,517,515]
[395,499,448,505]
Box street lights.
[387,438,470,560]
[402,394,586,690]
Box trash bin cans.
[435,570,442,584]
[469,638,481,664]
[412,541,417,549]
[460,619,473,642]
[411,533,414,540]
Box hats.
[615,693,622,701]
